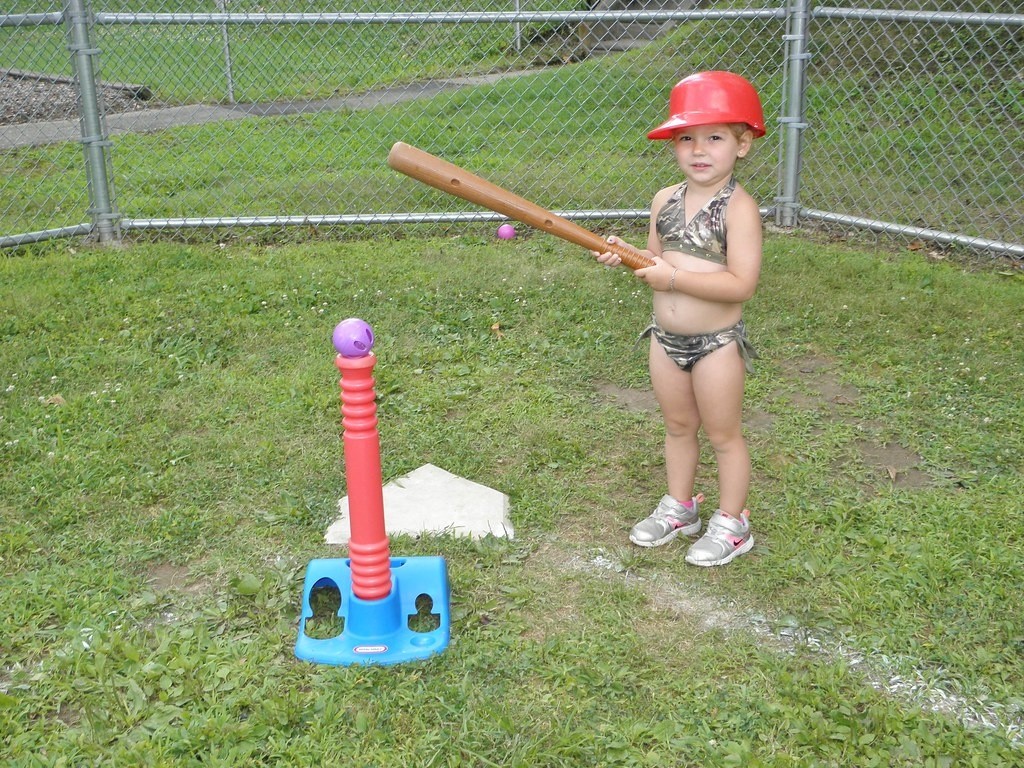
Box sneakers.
[628,493,704,547]
[685,509,754,566]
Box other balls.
[332,318,375,359]
[498,223,515,240]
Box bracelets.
[670,268,679,290]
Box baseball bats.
[387,140,658,269]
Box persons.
[592,69,769,567]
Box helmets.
[648,71,766,140]
